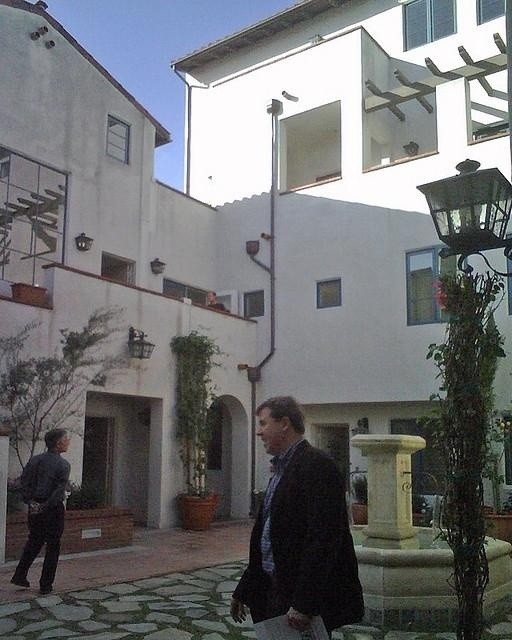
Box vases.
[177,494,219,531]
[10,283,48,305]
[483,514,511,540]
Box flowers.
[481,420,510,514]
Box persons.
[230,395,365,639]
[12,428,71,594]
[206,290,225,311]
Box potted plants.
[350,473,367,525]
[412,487,431,527]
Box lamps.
[350,417,369,436]
[128,326,154,360]
[75,232,93,251]
[402,140,419,157]
[137,408,150,432]
[416,157,511,278]
[150,257,166,273]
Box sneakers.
[10,578,30,587]
[40,586,52,594]
